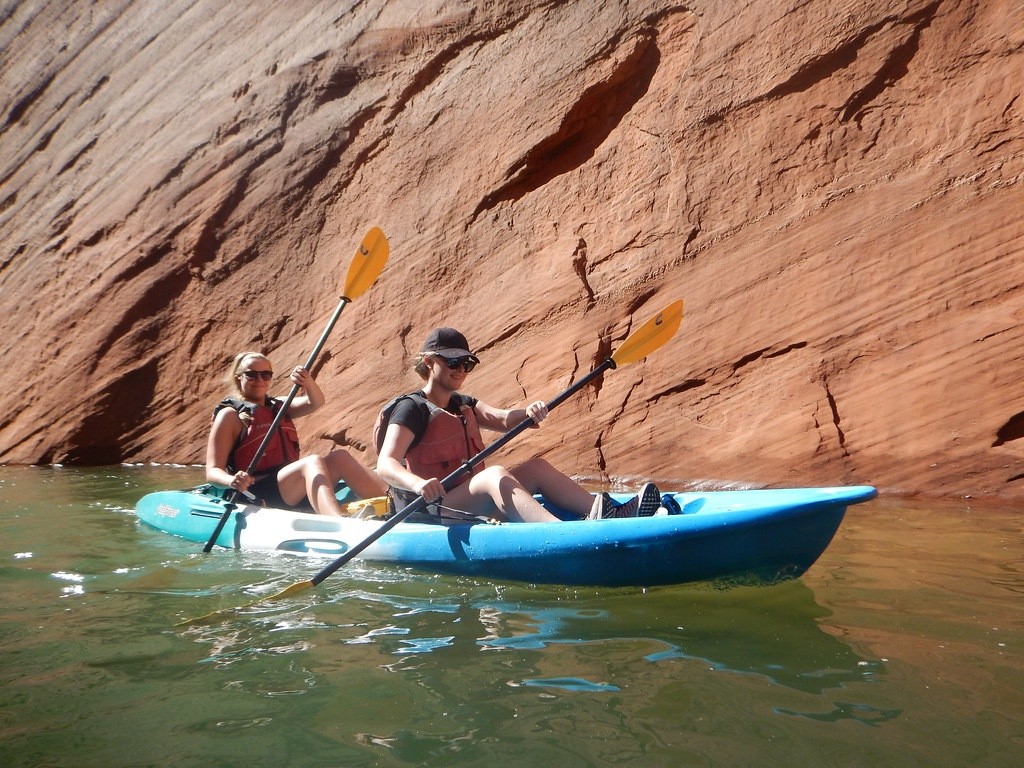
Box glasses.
[432,354,476,373]
[236,369,274,381]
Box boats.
[135,482,879,587]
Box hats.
[420,327,480,363]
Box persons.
[373,328,660,522]
[206,351,389,516]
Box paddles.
[201,225,391,557]
[267,295,687,604]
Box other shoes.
[348,503,375,520]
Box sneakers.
[578,492,615,520]
[612,482,661,518]
